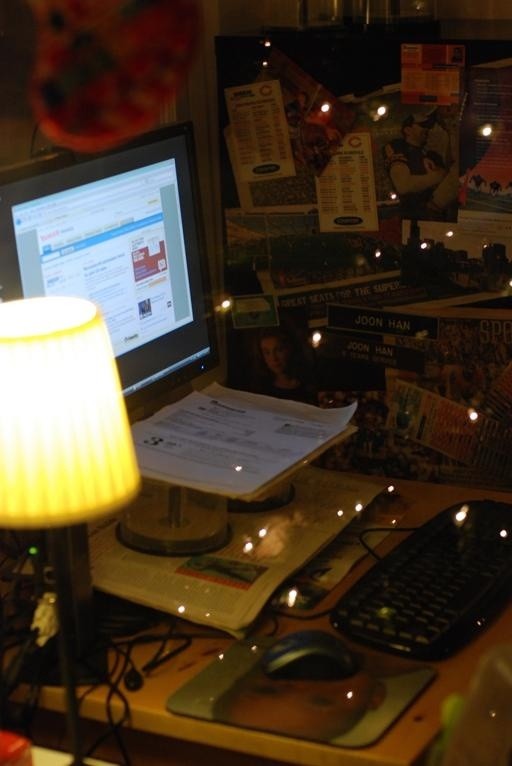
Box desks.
[0,462,511,766]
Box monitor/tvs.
[0,117,222,414]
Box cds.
[114,504,224,544]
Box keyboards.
[328,497,512,664]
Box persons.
[383,114,446,210]
[252,327,312,401]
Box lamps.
[0,296,132,688]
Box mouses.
[259,629,363,683]
